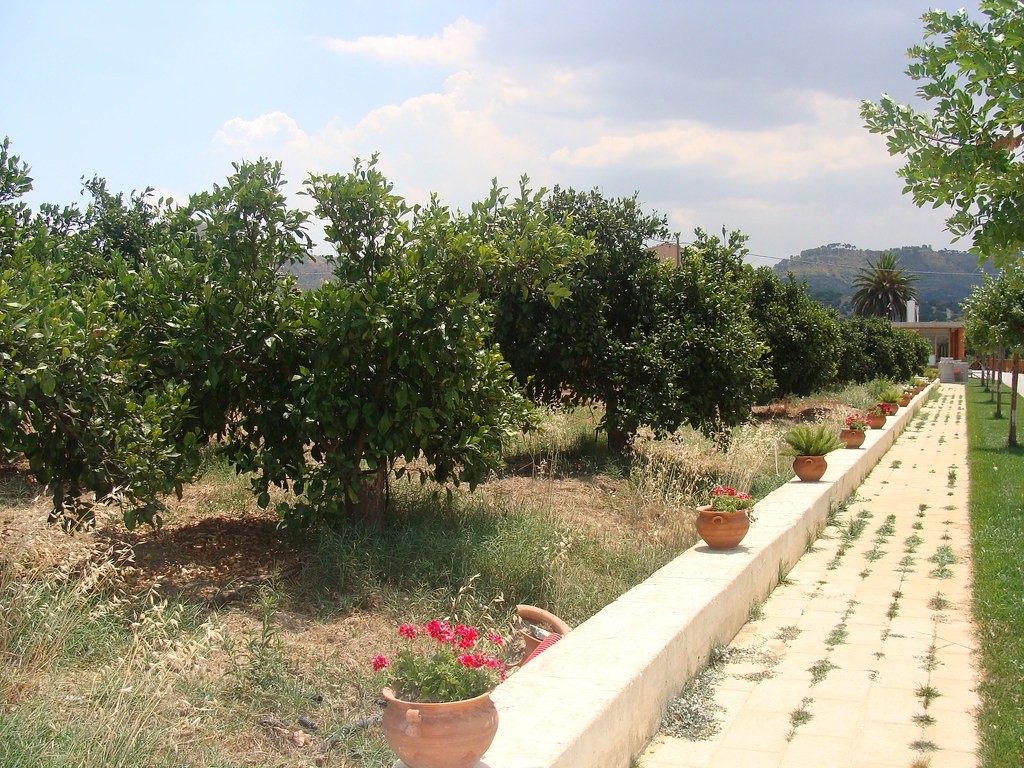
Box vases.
[840,428,866,449]
[955,375,959,381]
[867,415,887,429]
[380,685,499,768]
[514,604,570,668]
[695,505,750,550]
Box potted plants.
[903,378,927,399]
[879,389,900,414]
[778,421,846,482]
[898,393,914,407]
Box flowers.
[710,487,754,511]
[371,619,509,699]
[953,370,960,375]
[866,403,895,415]
[843,412,872,430]
